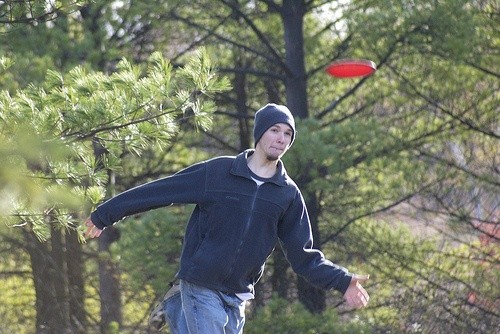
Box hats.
[253,104,295,148]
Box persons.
[83,104,370,334]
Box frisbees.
[325,61,376,78]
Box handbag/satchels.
[149,283,188,334]
